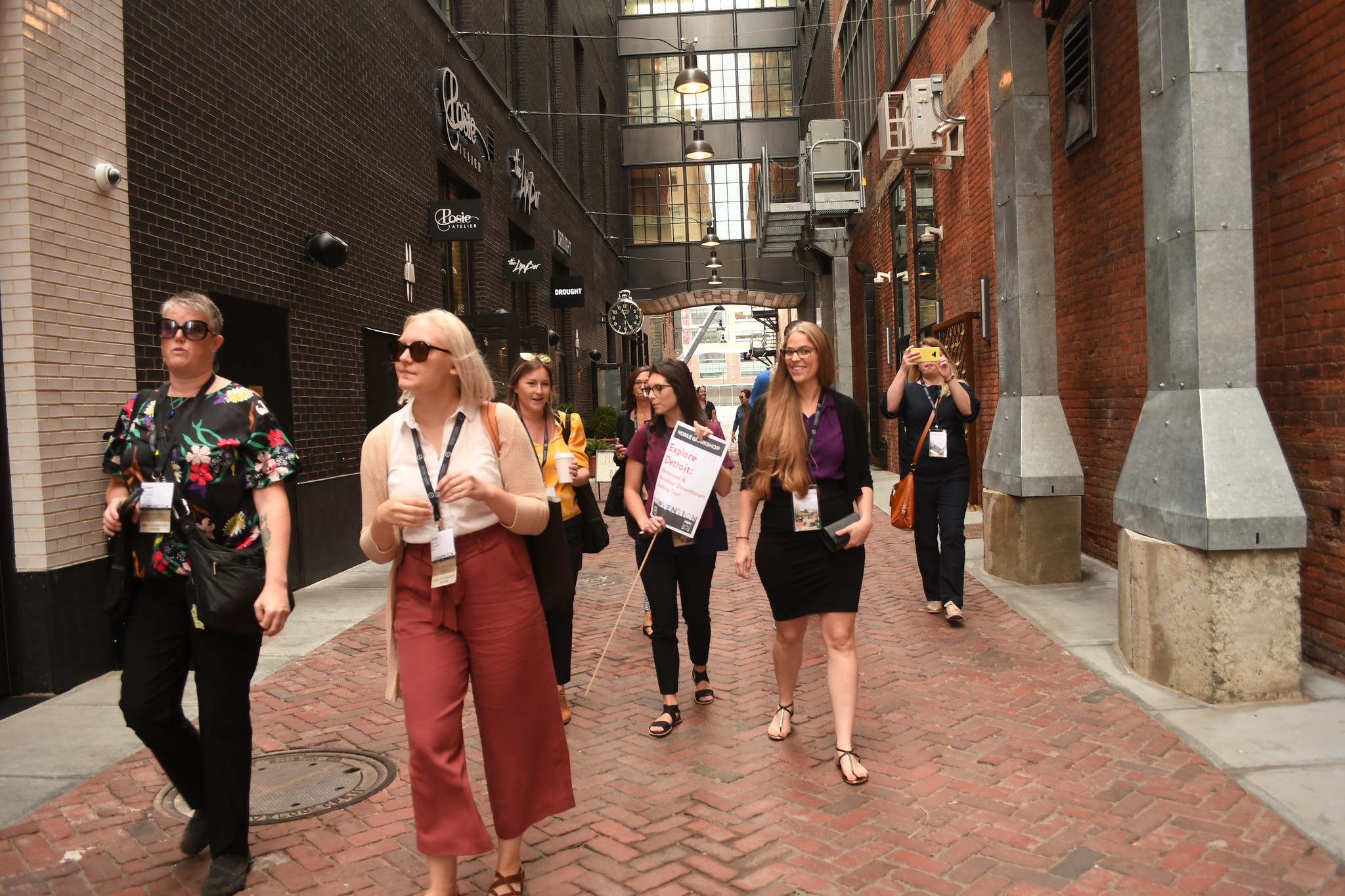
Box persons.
[734,321,873,784]
[498,351,590,725]
[102,291,299,896]
[731,389,752,491]
[360,305,576,896]
[623,361,736,739]
[613,366,657,640]
[880,336,980,619]
[696,386,719,424]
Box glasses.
[632,381,651,387]
[516,350,556,366]
[390,338,451,363]
[156,317,217,341]
[739,395,746,400]
[639,383,673,397]
[779,346,817,361]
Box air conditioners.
[904,78,943,151]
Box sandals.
[487,866,526,896]
[648,704,682,737]
[835,747,869,785]
[643,623,656,640]
[767,700,797,741]
[690,666,714,705]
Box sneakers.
[558,687,572,723]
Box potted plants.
[585,405,620,478]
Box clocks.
[598,288,645,337]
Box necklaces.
[637,409,651,421]
[742,406,746,416]
[167,384,201,419]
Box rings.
[618,452,622,456]
[456,484,461,492]
[947,359,949,363]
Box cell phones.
[118,488,145,516]
[910,347,940,362]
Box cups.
[554,453,574,484]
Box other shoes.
[926,601,943,614]
[200,852,253,896]
[180,809,209,854]
[944,601,964,619]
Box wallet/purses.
[820,511,861,552]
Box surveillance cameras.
[921,234,934,246]
[874,278,884,286]
[95,164,122,192]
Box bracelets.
[944,374,954,383]
[735,537,749,540]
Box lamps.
[507,108,715,160]
[582,211,724,285]
[443,25,714,96]
[663,304,728,345]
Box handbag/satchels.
[625,420,654,539]
[553,410,609,553]
[182,515,296,637]
[602,467,624,516]
[890,471,916,531]
